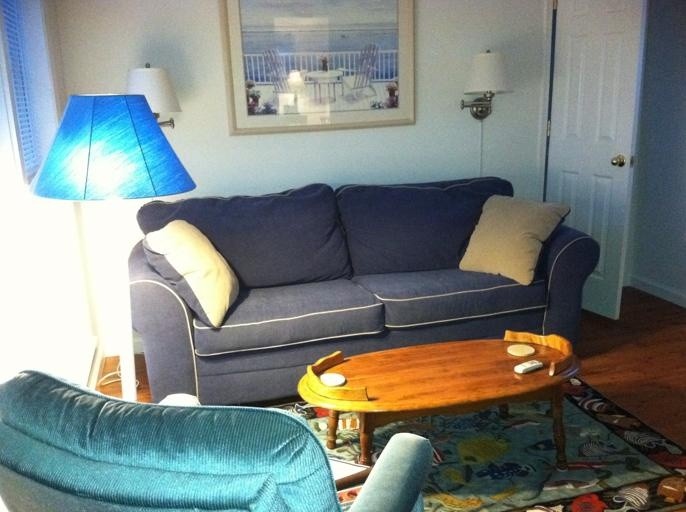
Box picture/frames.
[219,0,416,136]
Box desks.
[299,329,580,472]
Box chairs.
[0,370,431,512]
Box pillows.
[339,178,514,274]
[142,219,239,329]
[136,183,352,287]
[460,193,571,287]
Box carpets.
[242,370,686,512]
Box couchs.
[126,177,603,411]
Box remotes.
[514,359,543,374]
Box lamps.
[127,62,183,132]
[29,93,195,201]
[460,49,513,121]
